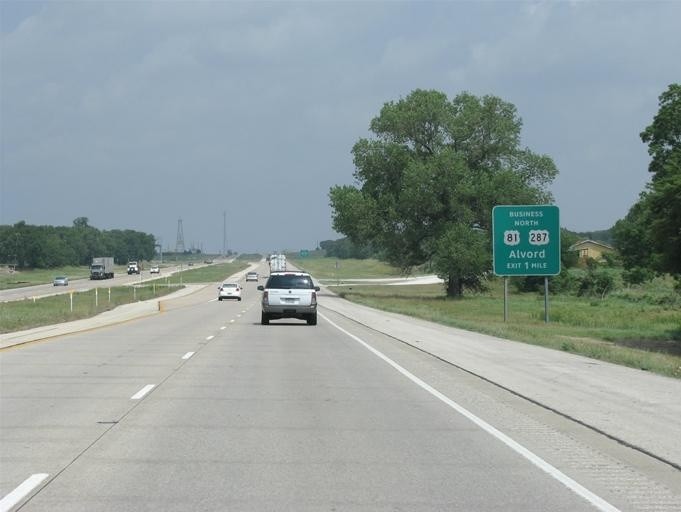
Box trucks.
[270,254,287,272]
[88,257,115,280]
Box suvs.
[258,271,320,325]
[127,261,140,275]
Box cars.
[54,276,68,286]
[245,272,258,280]
[218,283,243,301]
[150,266,160,274]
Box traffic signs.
[492,205,560,277]
